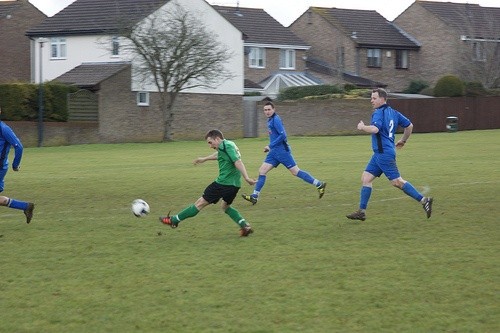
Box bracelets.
[401,140,406,143]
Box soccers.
[131,199,150,217]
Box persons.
[346,87,433,221]
[0,119,34,224]
[242,102,327,204]
[157,129,256,235]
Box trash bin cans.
[446,116,458,132]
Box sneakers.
[239,223,251,236]
[422,197,432,218]
[241,194,257,204]
[24,203,34,223]
[160,211,178,228]
[317,182,326,198]
[346,210,365,221]
